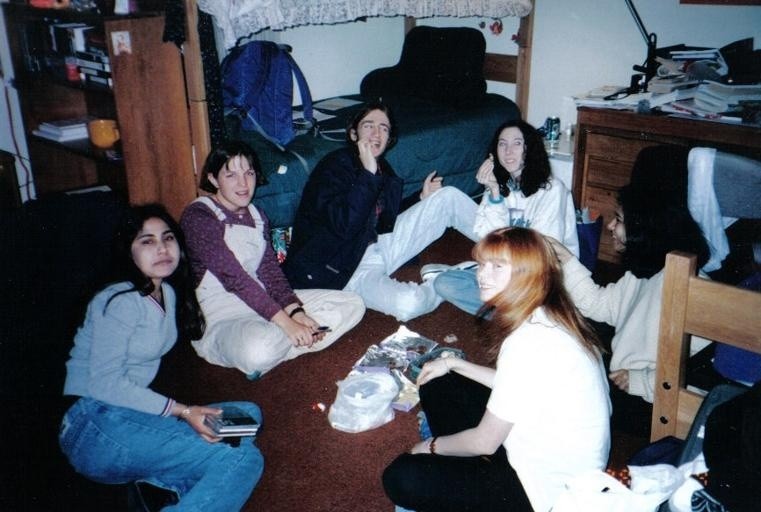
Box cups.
[88,118,122,151]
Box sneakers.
[420,260,480,281]
[417,411,432,440]
[127,482,178,512]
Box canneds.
[545,115,560,142]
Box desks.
[572,107,759,268]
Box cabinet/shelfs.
[578,132,657,264]
[4,6,198,209]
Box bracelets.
[289,306,306,318]
[430,435,439,454]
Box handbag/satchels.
[576,211,603,273]
[703,381,761,512]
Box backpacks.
[219,40,315,145]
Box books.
[206,404,261,438]
[573,48,761,123]
[31,117,89,143]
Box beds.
[181,1,534,232]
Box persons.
[178,140,366,379]
[436,120,582,320]
[281,104,481,327]
[59,202,267,511]
[387,228,614,510]
[557,169,709,458]
